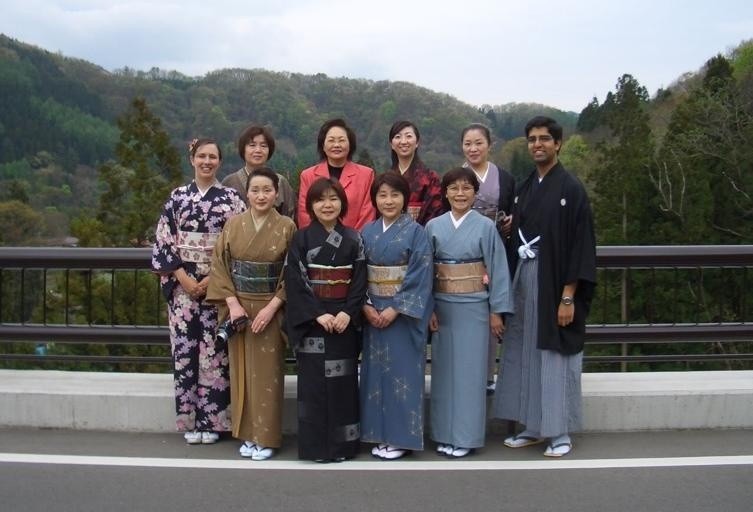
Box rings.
[261,321,264,323]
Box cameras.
[217,313,247,342]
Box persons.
[222,126,295,218]
[490,115,597,458]
[206,168,298,461]
[384,120,441,223]
[151,138,246,444]
[462,123,515,264]
[297,118,377,231]
[427,166,513,457]
[284,177,368,463]
[360,172,433,460]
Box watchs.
[560,295,575,306]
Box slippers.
[184,430,274,461]
[503,434,544,448]
[372,441,476,460]
[543,443,572,457]
